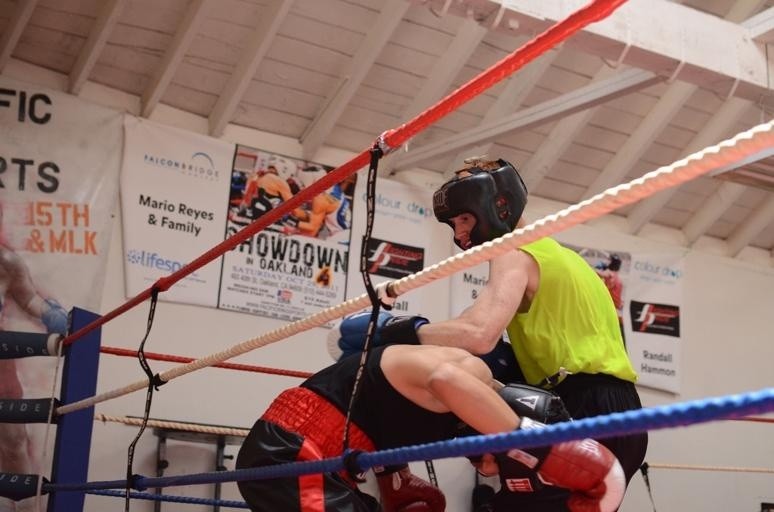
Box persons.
[235,334,625,512]
[0,198,69,512]
[594,254,624,331]
[287,166,352,237]
[237,172,308,221]
[326,156,649,512]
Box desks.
[123,415,254,512]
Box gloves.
[325,309,432,363]
[38,297,70,335]
[504,415,626,512]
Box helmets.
[257,170,265,176]
[268,154,296,181]
[499,382,570,424]
[432,157,529,250]
[298,166,328,188]
[607,254,621,271]
